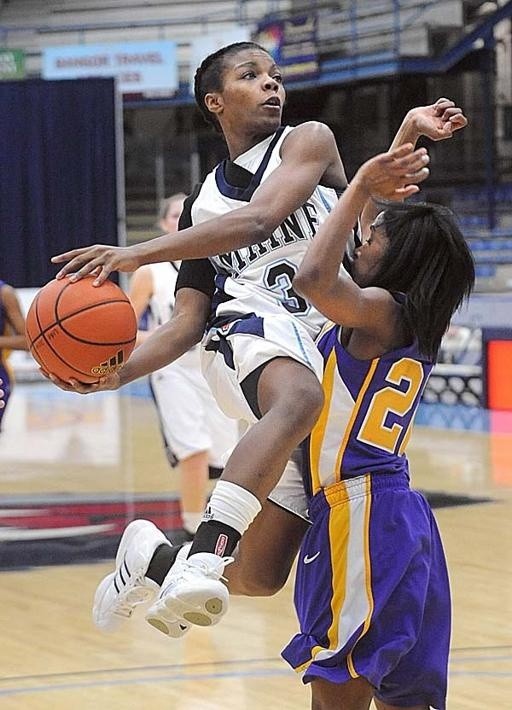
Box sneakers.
[144,552,229,638]
[91,518,173,635]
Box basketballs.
[25,273,137,385]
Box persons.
[282,92,477,710]
[130,191,256,541]
[0,279,29,433]
[48,34,366,640]
